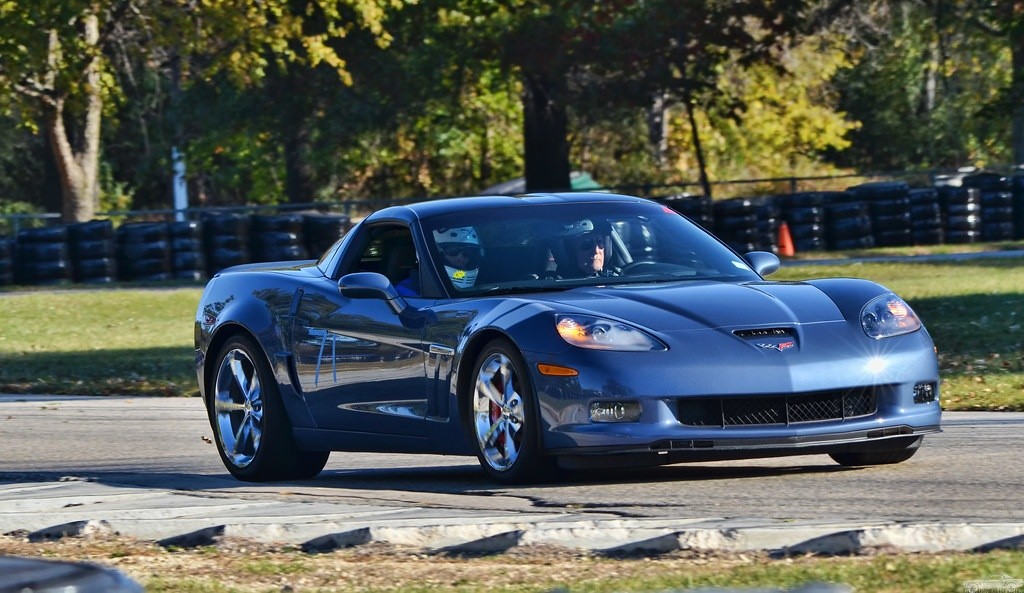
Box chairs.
[384,243,418,282]
[548,248,576,268]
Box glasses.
[440,245,477,258]
[581,238,604,251]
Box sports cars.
[191,192,946,484]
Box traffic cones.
[777,220,795,259]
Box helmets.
[555,212,614,274]
[432,224,484,289]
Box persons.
[557,215,624,281]
[394,224,480,299]
